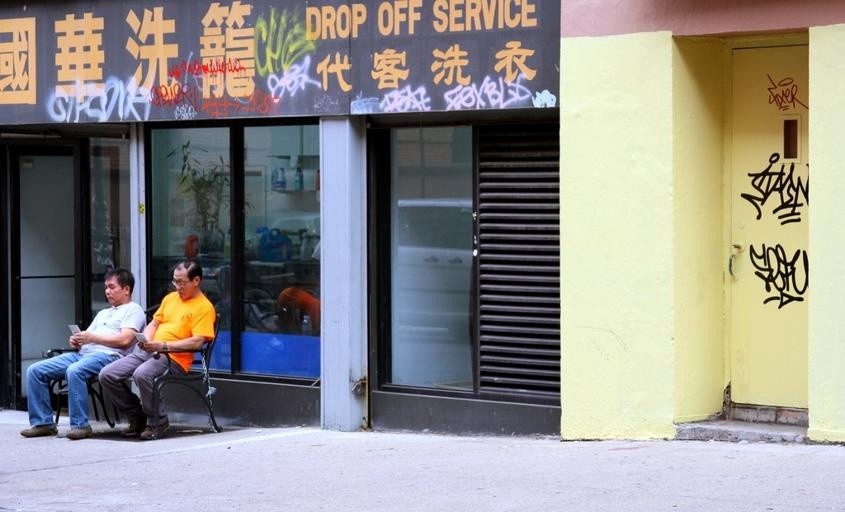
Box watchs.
[162,341,169,352]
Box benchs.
[47,313,226,437]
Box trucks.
[151,212,320,329]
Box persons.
[19,267,147,440]
[98,259,217,440]
[275,256,320,336]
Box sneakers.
[140,417,170,439]
[66,425,94,438]
[120,413,145,437]
[19,423,59,436]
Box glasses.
[171,279,191,290]
